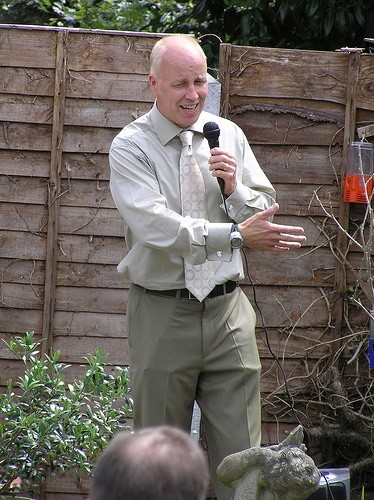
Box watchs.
[228,224,245,251]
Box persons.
[86,423,212,500]
[105,34,307,499]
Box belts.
[135,281,239,299]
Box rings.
[223,162,229,172]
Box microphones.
[203,121,225,194]
